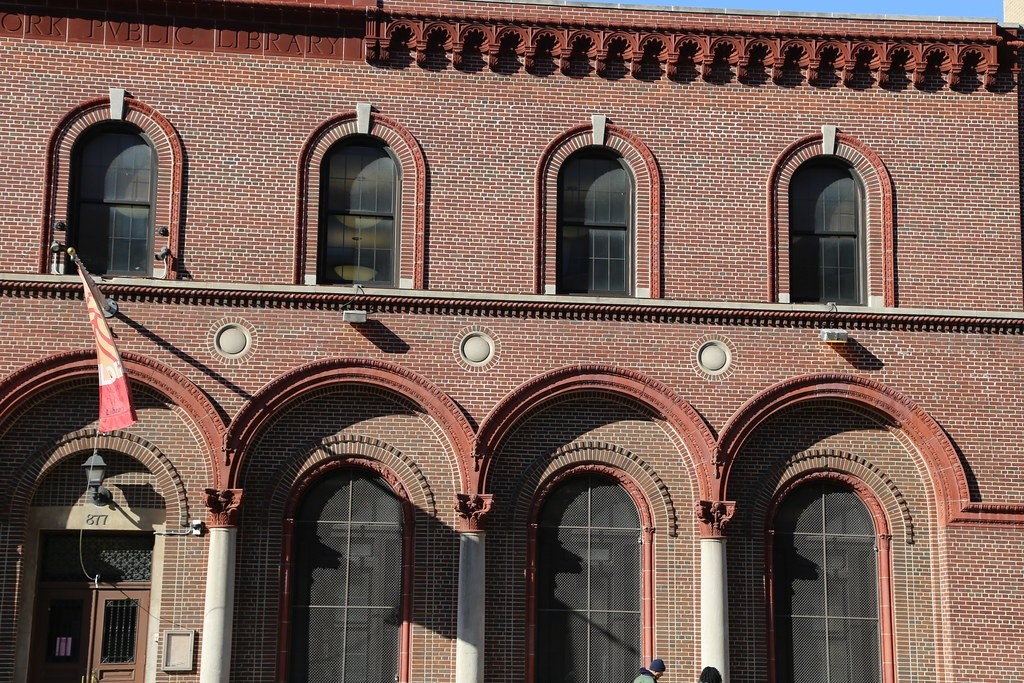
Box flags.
[69,247,139,432]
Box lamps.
[50,241,61,254]
[81,451,111,505]
[155,246,167,261]
[343,309,367,324]
[819,329,850,344]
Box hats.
[700,666,721,683]
[650,659,665,671]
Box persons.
[630,659,666,683]
[698,666,722,683]
